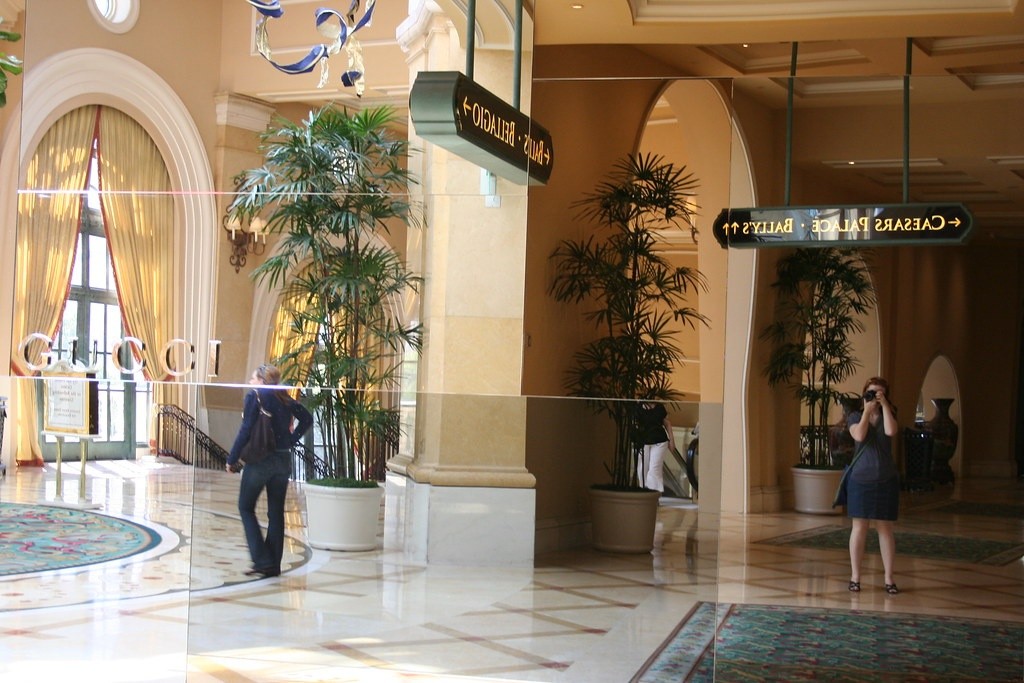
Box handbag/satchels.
[238,389,278,465]
[832,463,852,509]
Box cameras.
[863,390,877,402]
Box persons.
[227,364,316,575]
[845,378,903,594]
[633,390,675,491]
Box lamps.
[222,171,270,276]
[685,197,699,245]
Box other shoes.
[243,565,279,575]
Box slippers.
[884,584,898,594]
[848,581,860,591]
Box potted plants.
[230,100,427,552]
[549,148,713,556]
[766,243,888,515]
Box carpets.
[903,499,1024,521]
[0,501,163,576]
[754,523,1024,568]
[628,601,1024,683]
